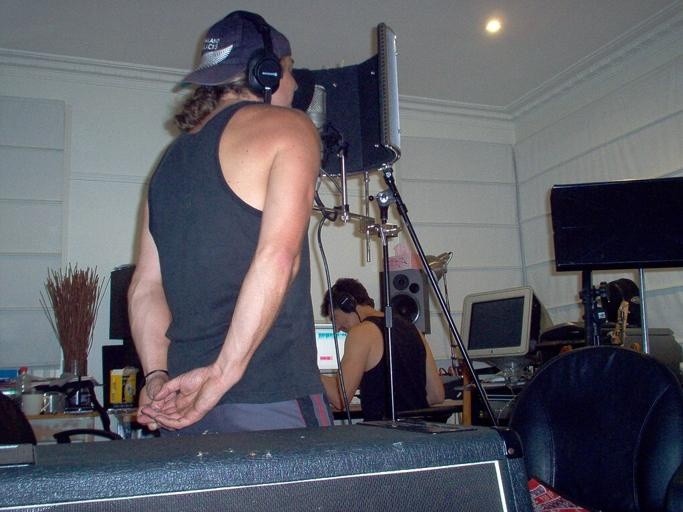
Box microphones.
[307,84,328,164]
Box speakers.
[0,419,534,512]
[383,269,431,333]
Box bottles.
[15,366,33,397]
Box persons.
[318,278,445,425]
[125,10,333,439]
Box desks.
[329,393,466,423]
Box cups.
[44,392,67,415]
[22,393,51,416]
[501,361,521,383]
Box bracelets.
[140,368,170,380]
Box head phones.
[236,10,281,96]
[332,285,357,314]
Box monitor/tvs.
[458,286,540,382]
[551,177,683,272]
[313,324,349,374]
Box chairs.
[501,345,683,510]
[1,391,128,444]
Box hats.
[184,13,291,86]
